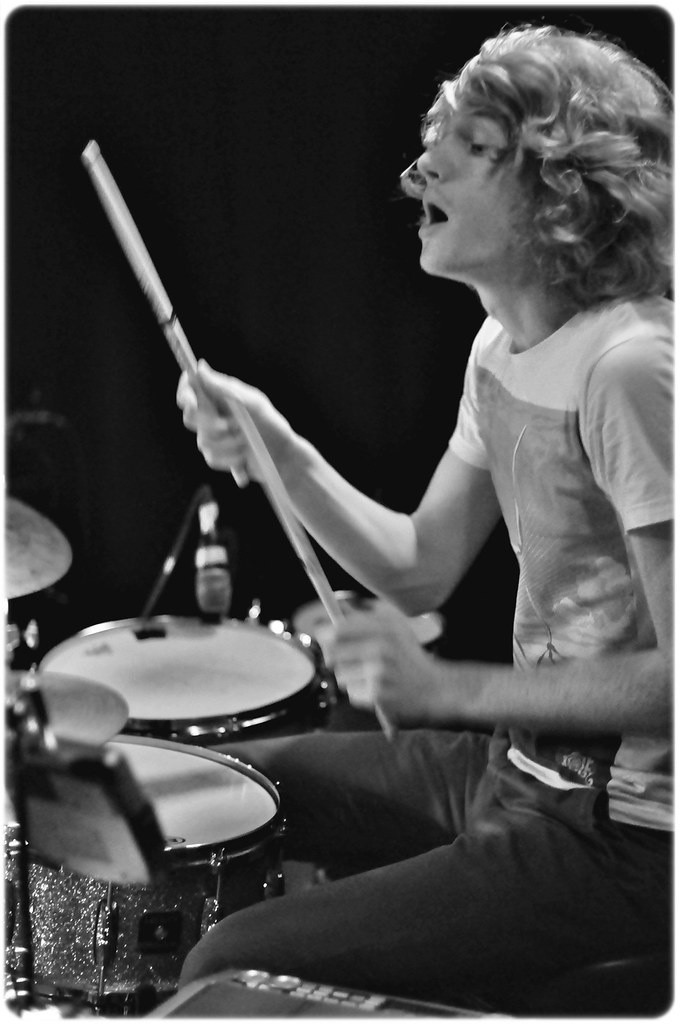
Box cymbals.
[4,495,131,762]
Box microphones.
[196,484,234,616]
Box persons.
[171,16,679,1022]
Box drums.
[7,590,337,1018]
